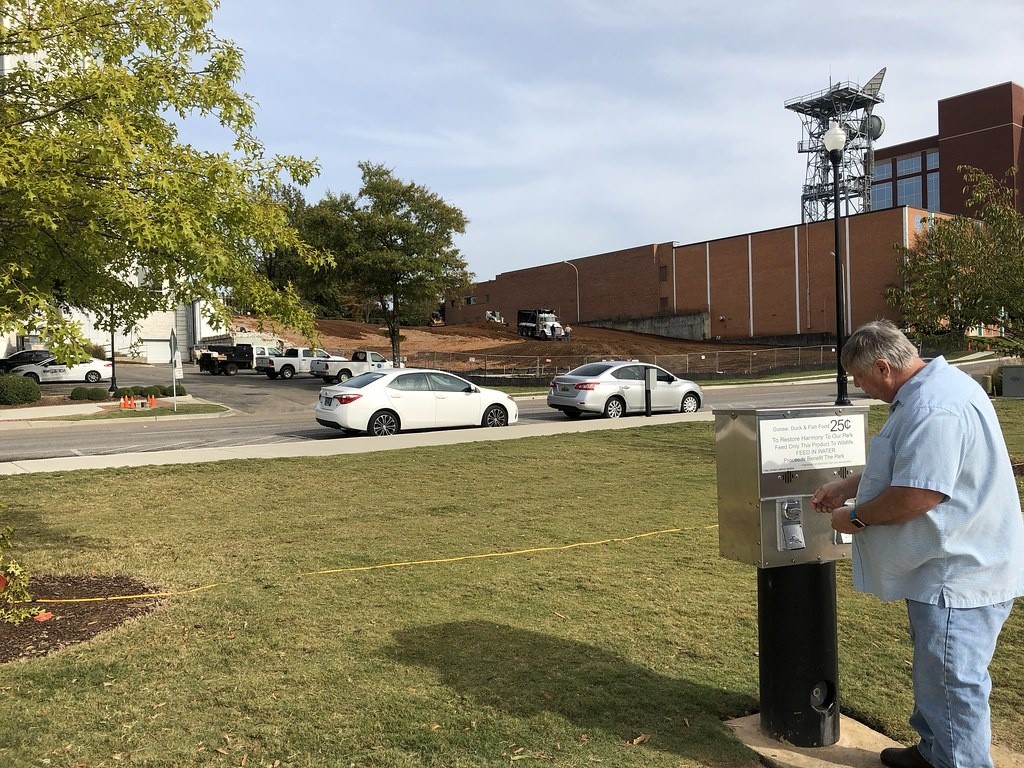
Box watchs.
[849,507,870,529]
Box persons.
[550,324,557,342]
[564,323,573,342]
[810,317,1024,768]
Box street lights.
[562,261,580,323]
[823,120,851,407]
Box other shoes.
[880,745,934,768]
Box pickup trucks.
[255,348,348,379]
[309,351,405,383]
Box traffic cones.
[119,394,134,409]
[147,394,156,407]
[967,342,971,351]
[986,344,991,351]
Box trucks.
[200,344,282,376]
[428,312,446,326]
[516,309,565,341]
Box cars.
[547,362,703,418]
[314,368,519,435]
[0,350,55,373]
[9,355,116,384]
